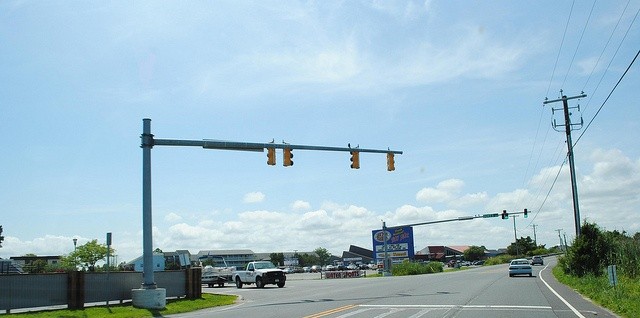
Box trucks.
[208,276,226,287]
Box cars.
[457,261,469,266]
[474,261,484,265]
[531,256,544,265]
[326,265,335,271]
[509,260,532,278]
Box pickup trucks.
[232,260,287,289]
[309,264,322,272]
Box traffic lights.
[386,152,395,171]
[524,209,527,218]
[283,149,293,166]
[350,151,358,169]
[267,147,276,165]
